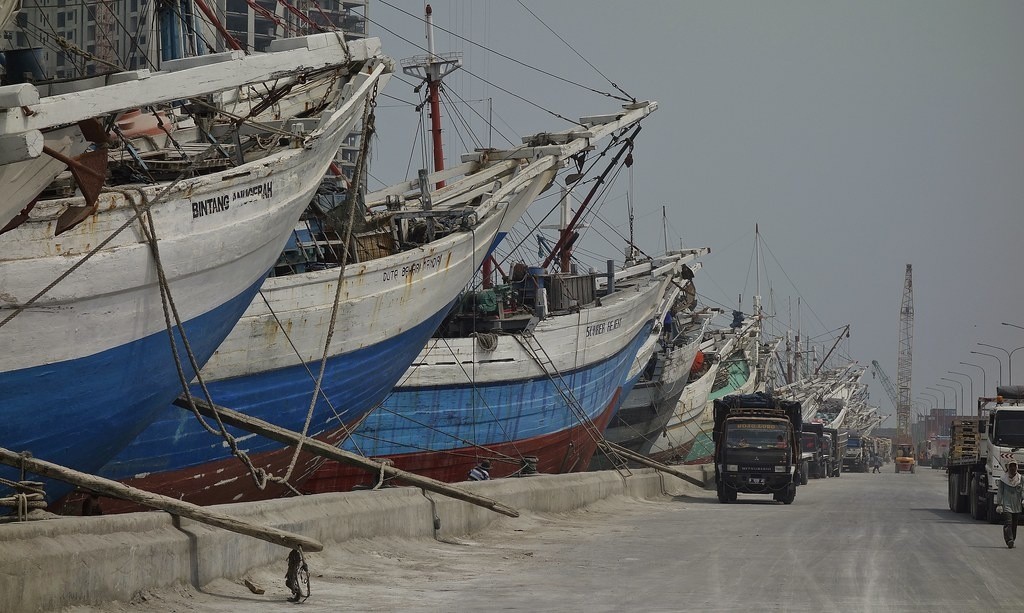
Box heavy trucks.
[946,385,1024,527]
[823,424,892,478]
[916,434,950,470]
[802,421,832,479]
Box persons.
[997,459,1024,549]
[467,460,493,481]
[873,452,881,473]
[897,446,913,458]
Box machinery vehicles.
[893,443,915,475]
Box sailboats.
[0,0,392,512]
[64,0,664,517]
[322,5,711,499]
[588,202,893,473]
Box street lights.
[1002,322,1024,329]
[960,362,985,398]
[935,383,957,416]
[977,342,1024,386]
[916,397,933,434]
[920,392,938,435]
[948,370,974,416]
[912,400,927,439]
[925,387,946,435]
[940,377,963,416]
[912,400,927,416]
[971,351,1002,387]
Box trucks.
[713,390,809,505]
